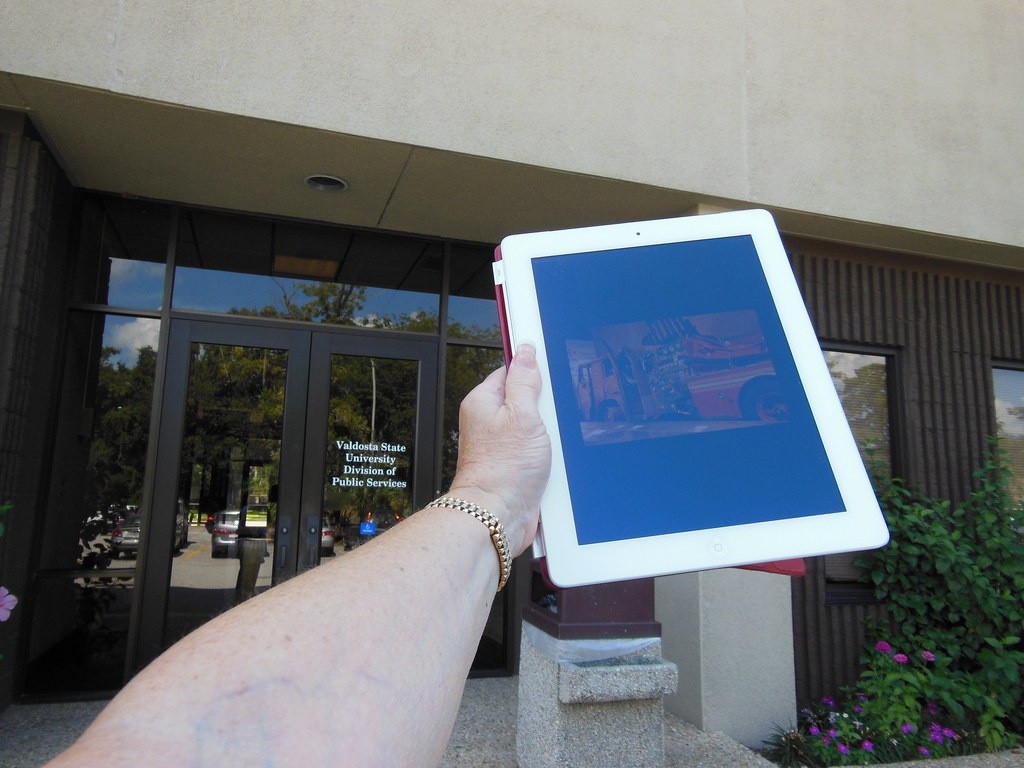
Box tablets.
[501,209,890,587]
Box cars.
[87,499,190,560]
[322,515,335,550]
[211,511,241,558]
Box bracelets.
[424,496,512,592]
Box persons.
[36,339,551,766]
